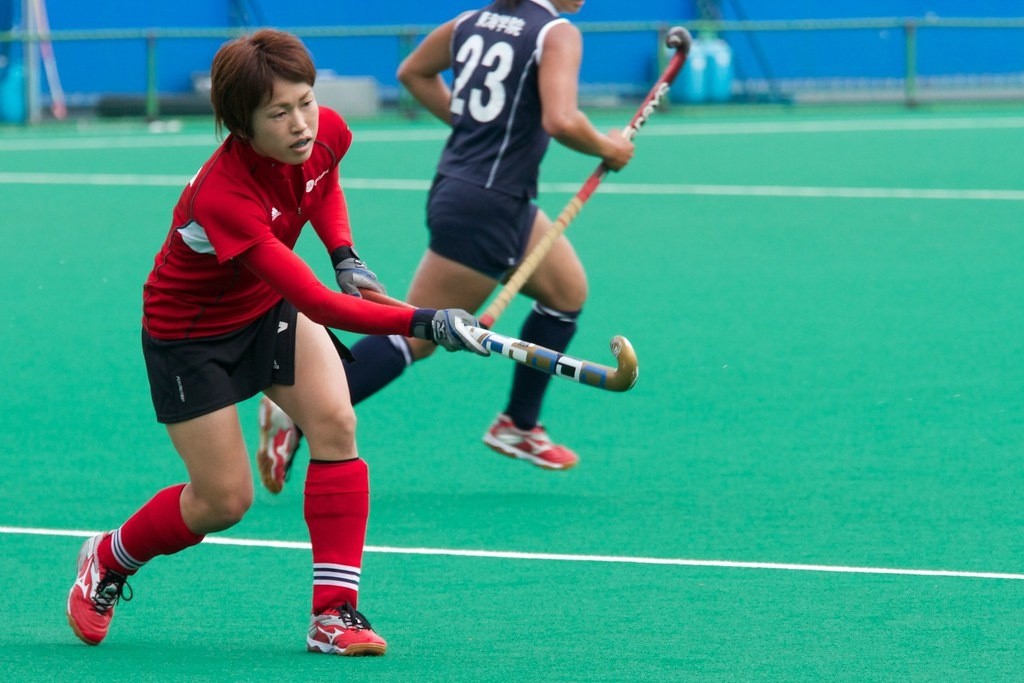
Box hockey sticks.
[359,286,640,391]
[478,26,695,331]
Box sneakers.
[257,397,299,494]
[67,530,133,645]
[306,603,386,655]
[483,412,579,470]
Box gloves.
[409,308,491,357]
[330,246,384,298]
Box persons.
[257,0,634,494]
[67,26,486,655]
